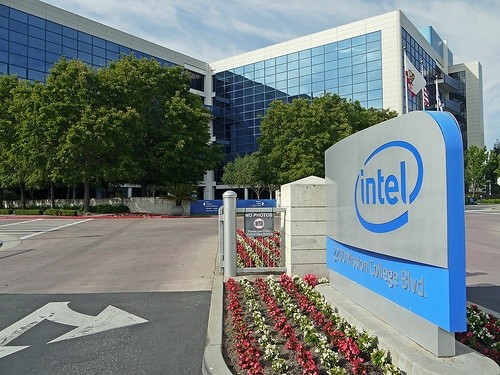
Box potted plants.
[158,175,199,216]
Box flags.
[435,81,443,112]
[424,87,430,106]
[405,55,427,97]
